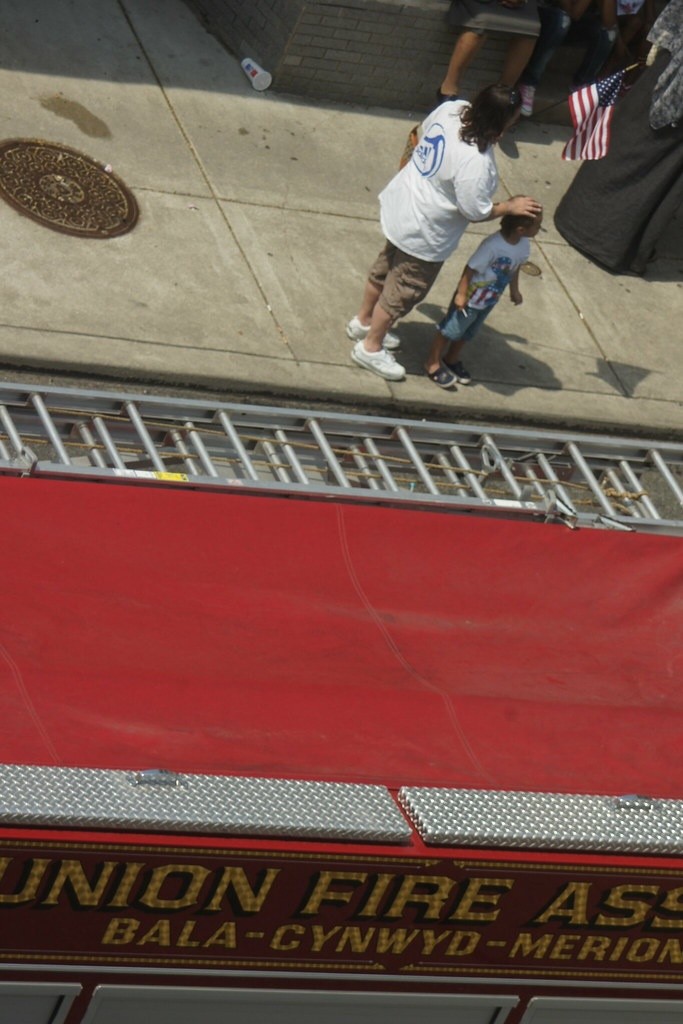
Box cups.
[242,57,273,91]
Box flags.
[560,71,625,162]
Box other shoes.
[423,360,457,388]
[436,86,459,105]
[441,354,471,384]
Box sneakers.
[350,340,406,380]
[347,313,400,349]
[517,83,536,117]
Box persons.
[517,0,632,118]
[346,80,543,382]
[424,195,544,390]
[437,0,543,105]
